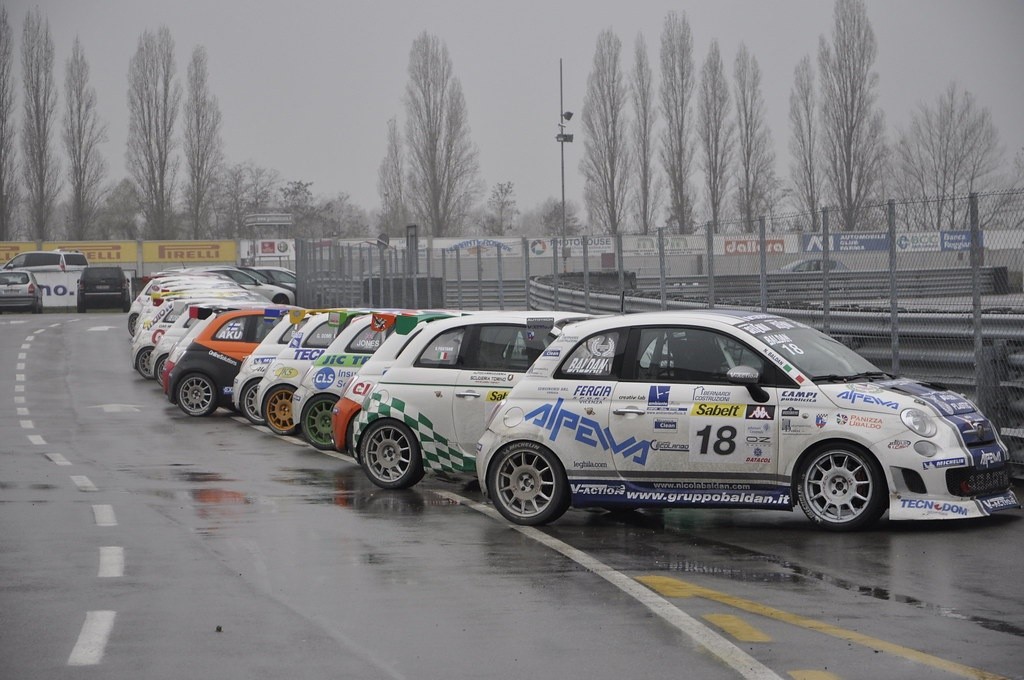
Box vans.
[1,248,88,271]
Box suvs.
[76,265,131,313]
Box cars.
[126,263,359,396]
[169,310,286,418]
[351,312,617,491]
[232,313,322,426]
[256,309,354,436]
[766,257,849,292]
[0,271,43,315]
[291,313,415,451]
[331,320,430,457]
[474,310,1023,534]
